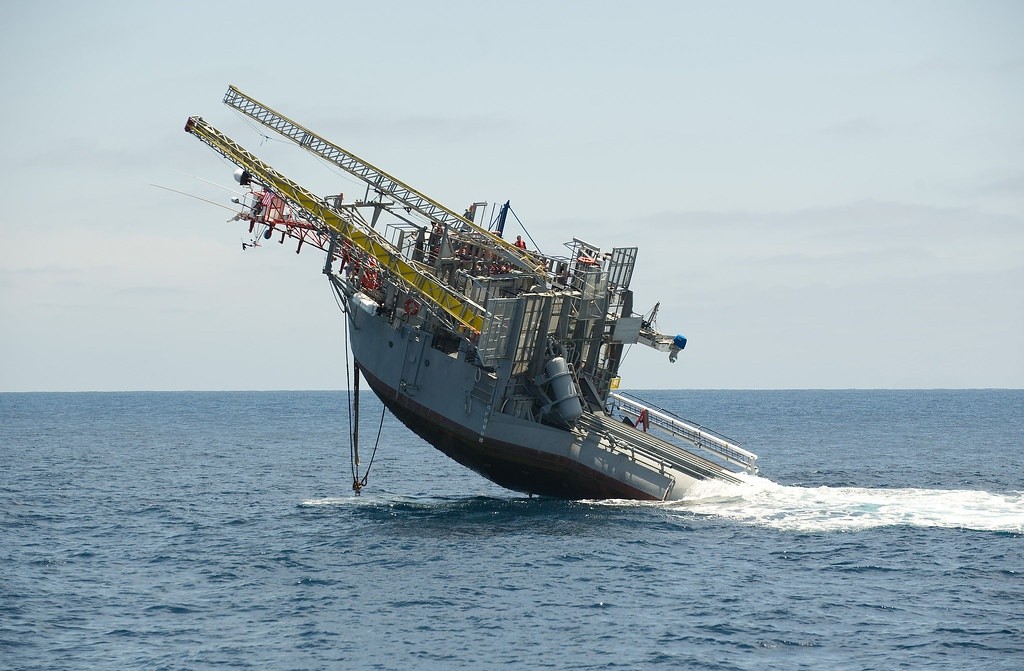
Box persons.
[515,236,526,249]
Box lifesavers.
[404,297,420,316]
[578,256,595,264]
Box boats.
[347,199,759,500]
